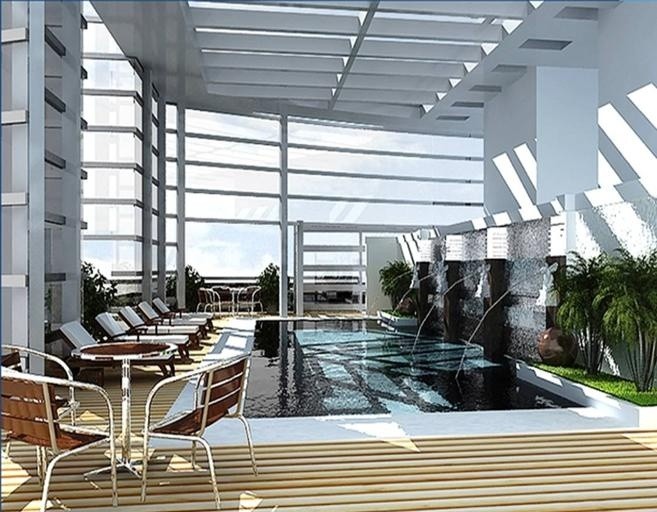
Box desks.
[71,341,177,482]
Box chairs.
[1,343,119,512]
[60,297,216,384]
[141,351,260,510]
[196,285,264,319]
